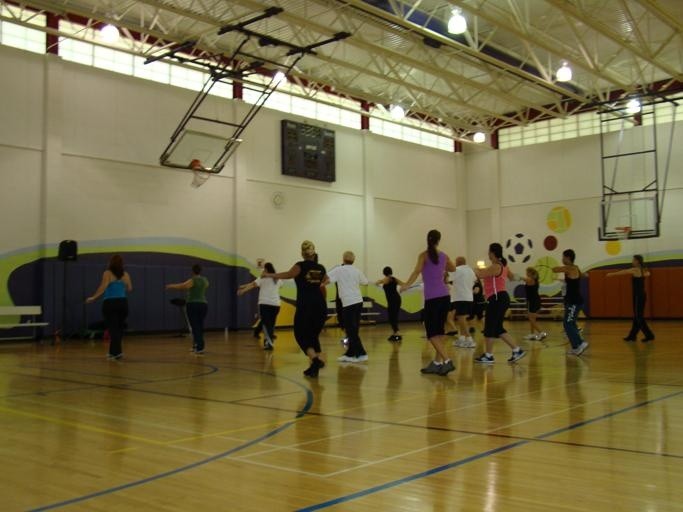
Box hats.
[301,240,316,257]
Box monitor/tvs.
[281,119,335,184]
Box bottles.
[55,328,60,344]
[56,344,61,352]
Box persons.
[321,249,369,363]
[606,254,656,343]
[260,239,329,379]
[560,356,587,444]
[379,347,405,413]
[239,275,279,340]
[324,261,351,345]
[415,376,457,510]
[374,265,406,343]
[628,344,657,426]
[466,275,485,334]
[334,366,375,456]
[518,265,548,342]
[448,255,479,351]
[399,229,457,375]
[287,381,335,497]
[100,366,132,466]
[85,254,133,361]
[552,248,590,356]
[477,368,519,458]
[313,253,328,300]
[250,353,282,443]
[525,347,548,408]
[446,280,459,336]
[163,264,210,354]
[188,359,212,441]
[474,243,527,364]
[236,261,282,351]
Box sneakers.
[475,352,495,363]
[623,336,654,343]
[523,329,589,355]
[422,362,454,376]
[446,325,475,347]
[107,352,123,360]
[387,335,402,341]
[253,334,277,350]
[304,359,324,376]
[336,354,368,362]
[507,349,526,362]
[190,345,205,354]
